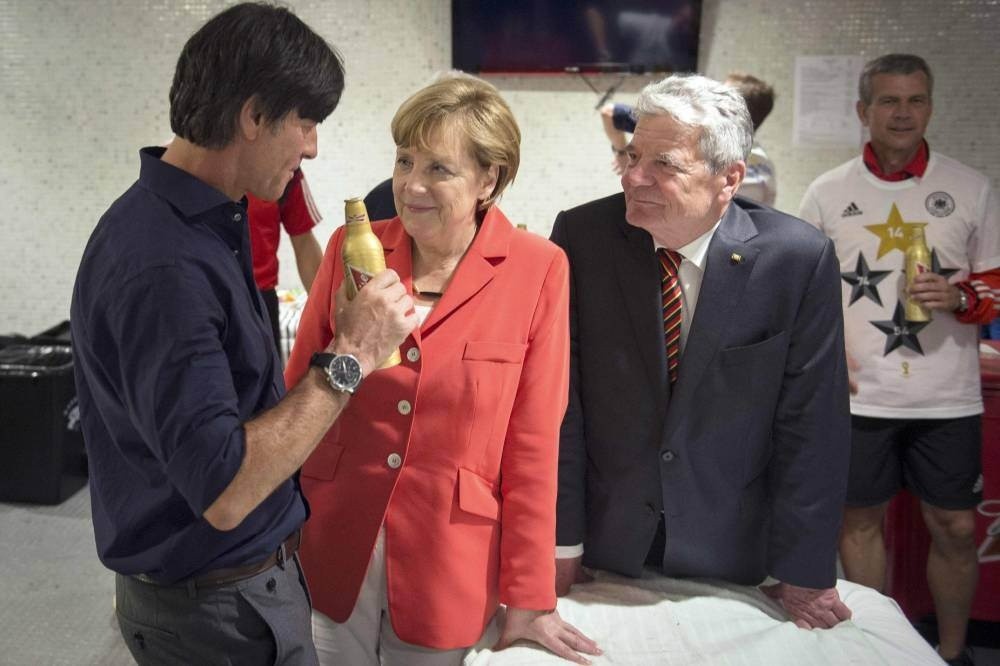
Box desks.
[460,568,950,666]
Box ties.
[656,247,686,396]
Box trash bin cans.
[0,344,89,504]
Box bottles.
[905,226,933,322]
[341,198,404,369]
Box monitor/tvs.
[451,1,701,77]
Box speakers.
[0,341,90,507]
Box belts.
[132,526,302,589]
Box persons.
[281,68,603,666]
[600,71,776,207]
[363,177,398,221]
[549,66,852,634]
[797,52,1000,666]
[245,165,327,358]
[70,0,423,666]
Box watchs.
[309,351,363,396]
[955,289,967,314]
